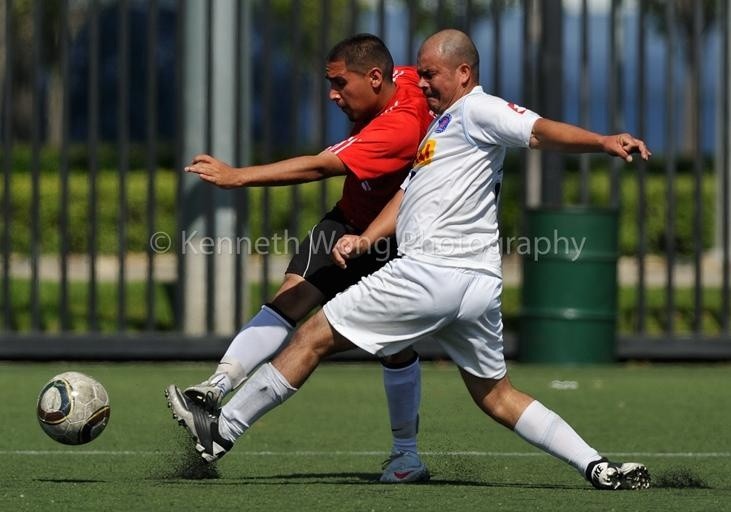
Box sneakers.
[380,452,430,485]
[585,456,651,491]
[164,383,235,462]
[183,372,233,411]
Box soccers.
[36,372,110,445]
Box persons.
[157,27,655,489]
[182,30,438,485]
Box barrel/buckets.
[518,204,617,365]
